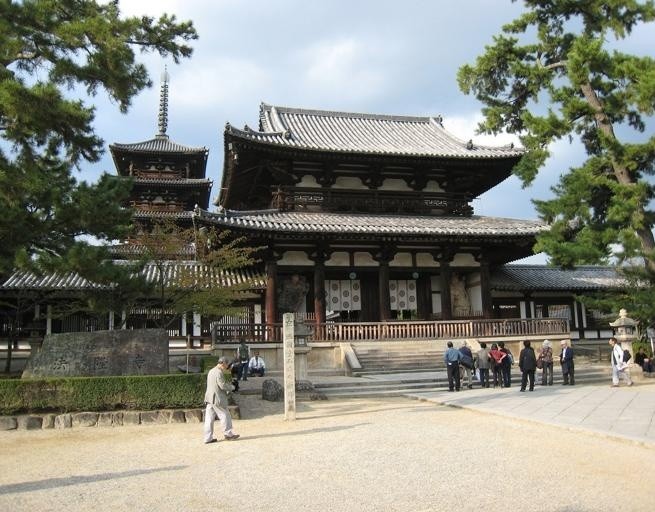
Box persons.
[456,340,473,389]
[609,337,634,388]
[475,343,491,388]
[488,343,507,388]
[497,341,514,387]
[203,356,240,444]
[538,339,553,386]
[635,346,655,373]
[518,340,536,391]
[444,341,464,392]
[558,339,576,386]
[226,338,265,385]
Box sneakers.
[205,438,218,443]
[224,433,241,440]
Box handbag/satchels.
[459,354,475,369]
[535,354,545,370]
[622,349,631,363]
